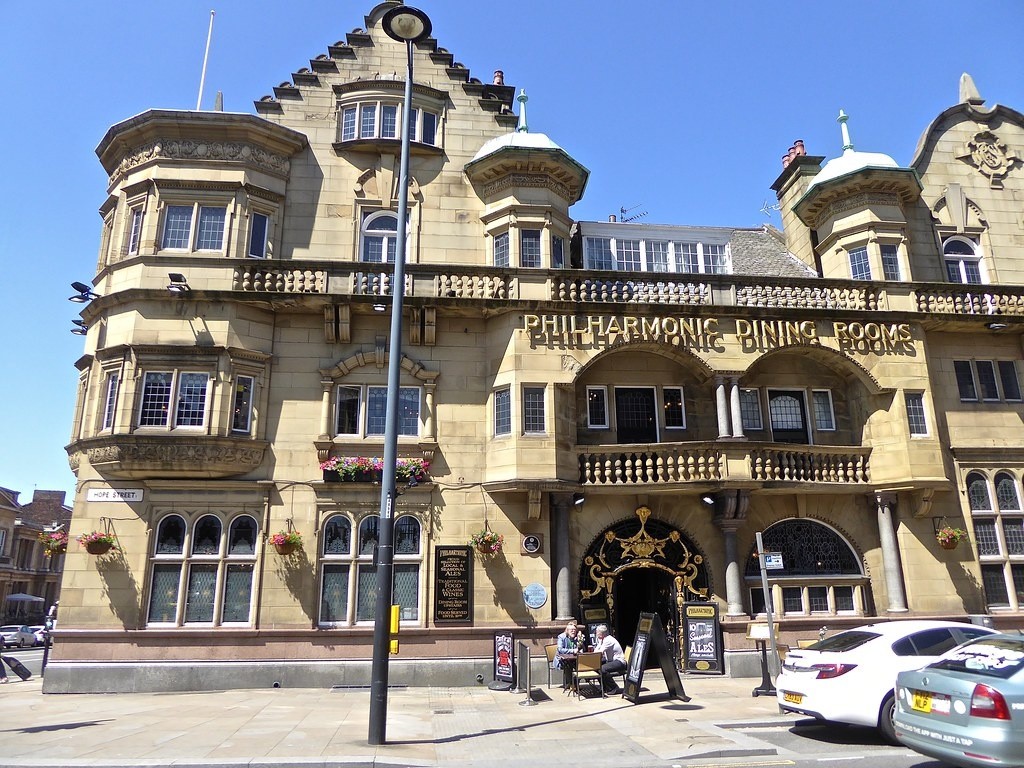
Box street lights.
[366,7,432,743]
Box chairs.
[610,645,634,686]
[545,644,567,689]
[573,652,605,701]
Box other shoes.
[604,686,619,695]
[570,684,576,690]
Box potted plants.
[1018,591,1024,601]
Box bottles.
[573,639,577,656]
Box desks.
[558,652,605,697]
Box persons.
[553,623,584,691]
[593,625,627,695]
[0,634,8,683]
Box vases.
[323,469,426,482]
[476,542,495,553]
[942,541,959,551]
[275,542,296,554]
[85,542,112,554]
[48,544,67,555]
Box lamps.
[372,302,387,313]
[166,274,191,298]
[984,323,1008,331]
[573,492,585,512]
[70,320,88,335]
[69,282,101,303]
[700,493,718,505]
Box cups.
[588,646,593,652]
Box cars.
[776,618,1003,747]
[1,625,37,648]
[896,631,1024,768]
[28,626,53,647]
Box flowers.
[76,530,126,555]
[268,530,303,554]
[465,530,504,564]
[319,454,431,482]
[936,527,970,549]
[39,530,69,560]
